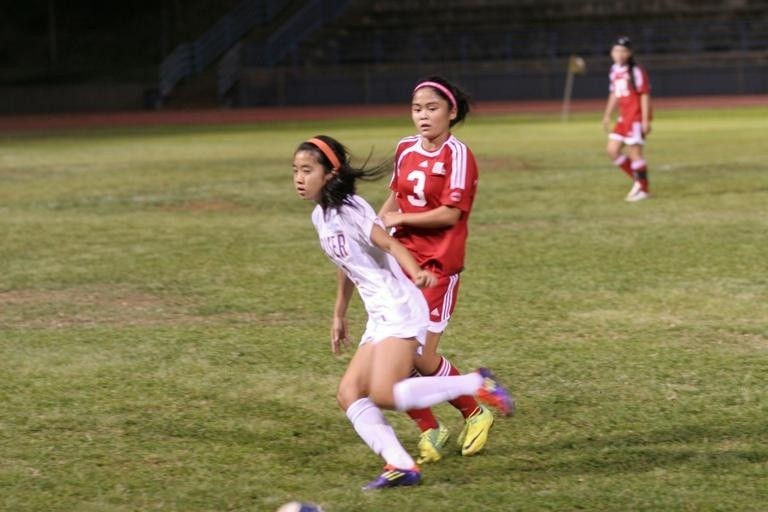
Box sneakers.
[416,419,450,464]
[474,367,515,418]
[362,465,420,490]
[624,179,649,203]
[461,407,495,456]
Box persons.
[601,35,654,203]
[291,135,517,491]
[375,74,495,465]
